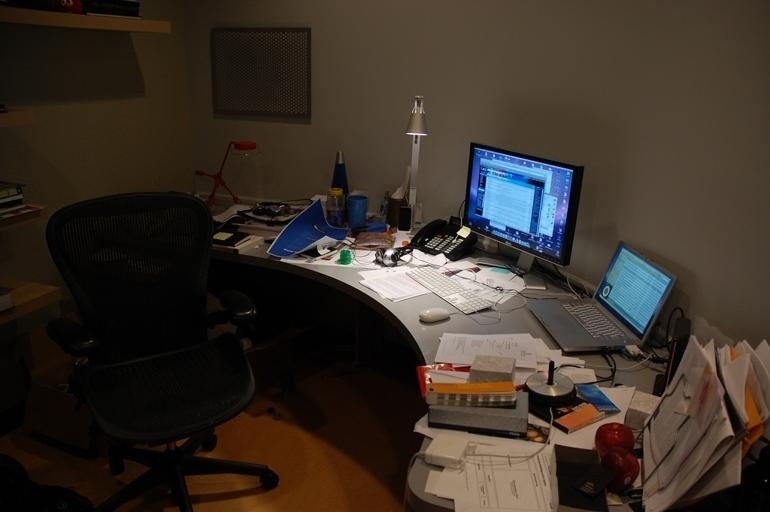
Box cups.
[346,195,368,227]
[386,196,407,227]
[595,421,640,493]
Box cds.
[527,371,574,397]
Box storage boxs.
[24,362,95,451]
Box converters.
[425,436,467,467]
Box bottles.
[332,150,350,195]
[326,188,346,228]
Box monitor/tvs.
[463,142,585,291]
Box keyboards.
[406,266,493,315]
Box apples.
[596,421,640,490]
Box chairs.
[44,189,281,511]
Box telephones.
[409,219,478,261]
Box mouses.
[419,309,449,323]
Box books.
[548,443,609,512]
[0,182,45,226]
[513,381,605,434]
[211,230,264,256]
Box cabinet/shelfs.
[0,200,66,327]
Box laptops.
[526,241,678,353]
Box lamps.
[193,139,257,206]
[402,95,429,229]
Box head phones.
[375,247,413,267]
[254,202,286,215]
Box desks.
[208,203,678,511]
